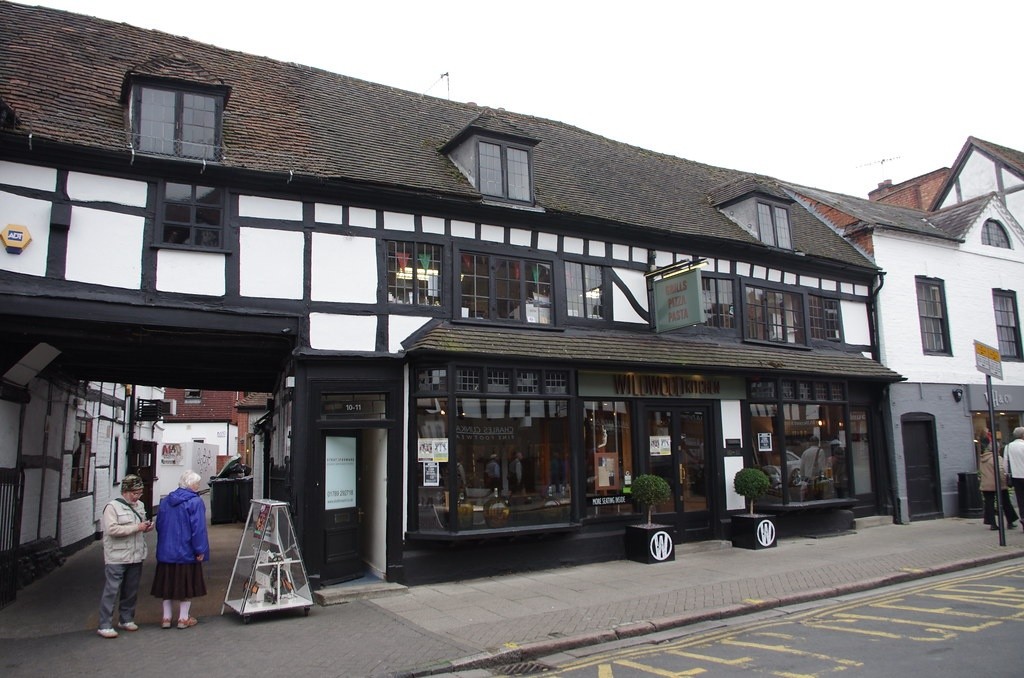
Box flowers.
[482,496,510,516]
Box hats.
[121,474,145,495]
[490,454,497,459]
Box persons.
[508,452,527,496]
[486,454,500,480]
[457,456,466,489]
[980,430,1019,530]
[828,447,847,481]
[285,456,290,472]
[800,436,825,482]
[96,473,154,638]
[1003,426,1024,532]
[149,472,210,629]
[223,461,245,477]
[270,457,274,465]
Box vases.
[482,512,508,528]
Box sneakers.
[178,614,198,629]
[118,622,138,631]
[161,616,171,628]
[97,626,118,638]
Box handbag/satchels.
[800,480,808,503]
[813,448,821,477]
[1006,473,1013,488]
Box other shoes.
[991,525,999,530]
[1007,522,1018,529]
[983,518,991,524]
[504,501,511,507]
[524,498,532,503]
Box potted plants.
[624,474,675,565]
[730,468,778,551]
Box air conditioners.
[161,398,177,415]
